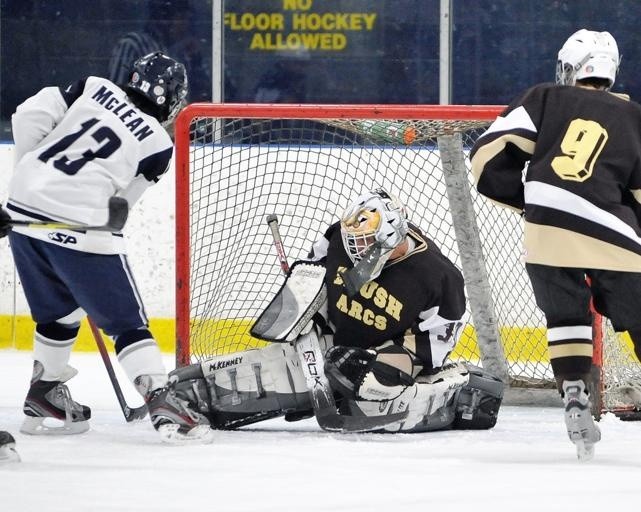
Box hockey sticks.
[266,215,409,433]
[85,314,149,422]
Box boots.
[562,378,602,444]
[145,388,211,433]
[24,359,92,422]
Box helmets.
[125,50,188,119]
[558,28,620,90]
[339,188,409,265]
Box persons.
[4,50,211,440]
[170,188,504,439]
[467,24,639,443]
